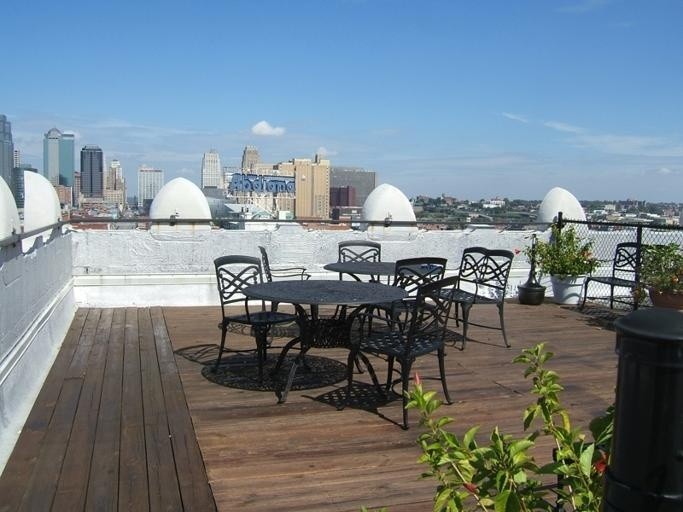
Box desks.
[238,280,410,404]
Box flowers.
[515,220,599,274]
[399,342,618,512]
[640,243,683,293]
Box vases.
[650,290,683,314]
[518,275,589,308]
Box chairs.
[336,276,460,429]
[212,255,314,380]
[581,242,656,313]
[258,239,514,351]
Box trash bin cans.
[598,308,683,512]
